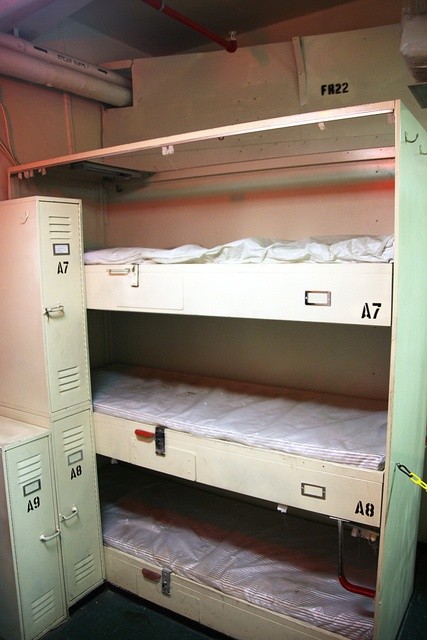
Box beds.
[84,234,393,326]
[96,453,379,640]
[91,361,388,527]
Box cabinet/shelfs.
[0,429,66,640]
[0,196,93,421]
[53,410,105,608]
